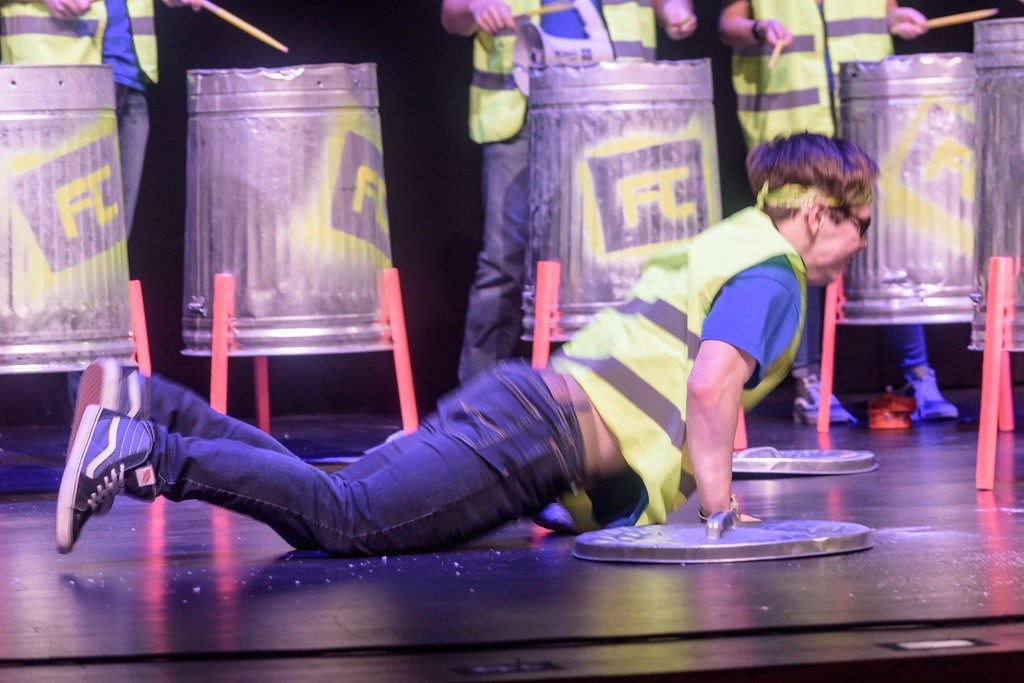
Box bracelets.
[753,21,759,39]
[698,496,742,522]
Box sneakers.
[64,356,143,467]
[55,403,158,554]
[793,374,850,425]
[898,370,956,421]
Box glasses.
[826,206,872,236]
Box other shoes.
[958,404,981,430]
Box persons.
[440,0,697,384]
[1,0,204,431]
[56,132,878,553]
[719,0,958,422]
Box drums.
[518,52,726,343]
[0,56,141,382]
[831,50,980,330]
[180,59,401,360]
[964,13,1023,355]
